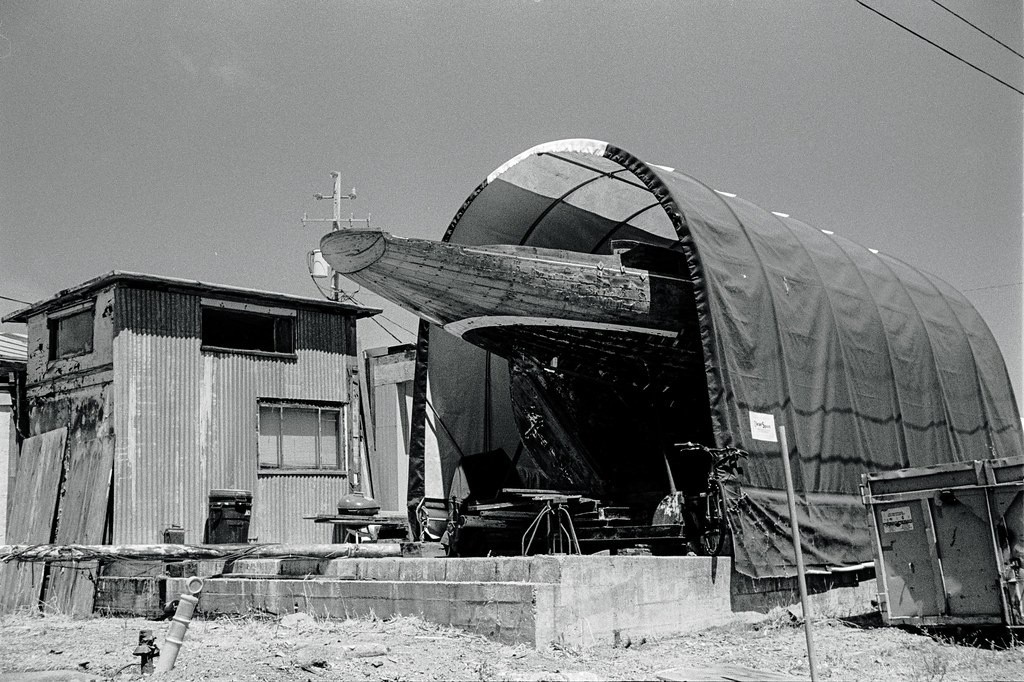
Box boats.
[316,227,704,387]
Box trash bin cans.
[207,488,254,544]
[856,452,1024,634]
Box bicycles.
[673,441,749,556]
